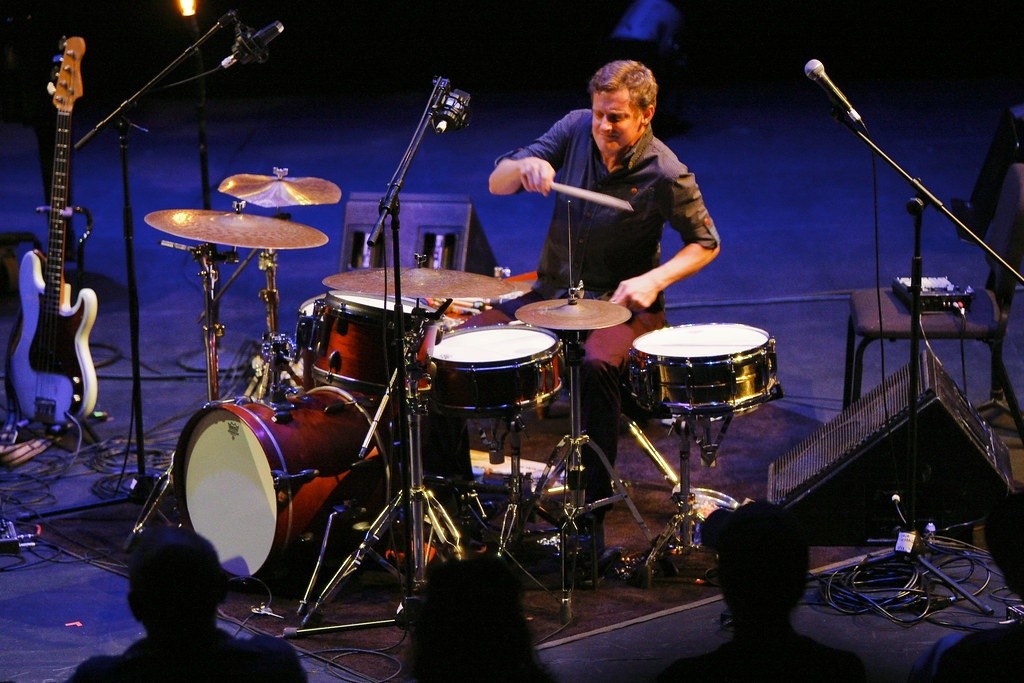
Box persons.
[427,60,721,560]
[657,501,866,683]
[402,561,559,683]
[60,527,308,683]
[906,493,1024,683]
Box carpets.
[15,402,893,682]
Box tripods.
[247,79,715,641]
[806,116,1024,615]
[17,8,237,525]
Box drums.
[172,384,394,585]
[626,323,781,422]
[421,324,567,418]
[304,291,442,395]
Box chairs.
[844,162,1024,447]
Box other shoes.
[567,519,605,553]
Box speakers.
[766,349,1015,548]
[338,193,503,283]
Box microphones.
[218,22,284,69]
[436,88,471,133]
[805,59,865,129]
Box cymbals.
[217,172,343,210]
[143,209,330,251]
[513,298,633,331]
[322,266,517,299]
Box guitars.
[4,36,100,426]
